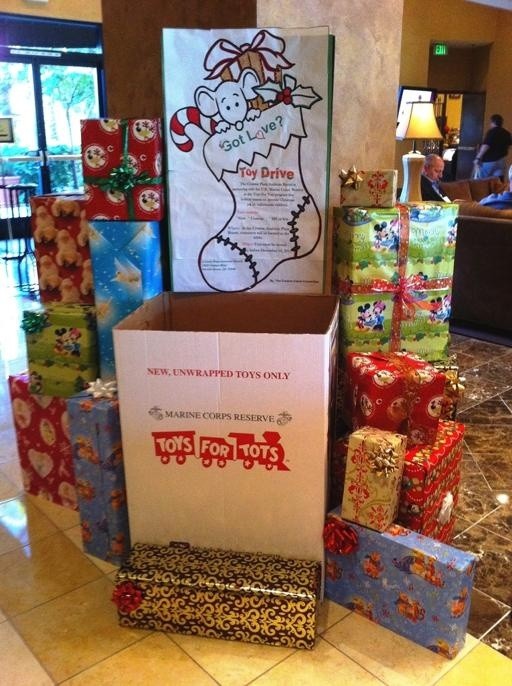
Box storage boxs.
[111,288,343,557]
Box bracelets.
[474,157,480,161]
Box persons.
[448,137,456,144]
[477,163,512,209]
[421,153,452,202]
[473,114,512,183]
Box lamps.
[394,99,445,202]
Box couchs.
[434,177,510,347]
